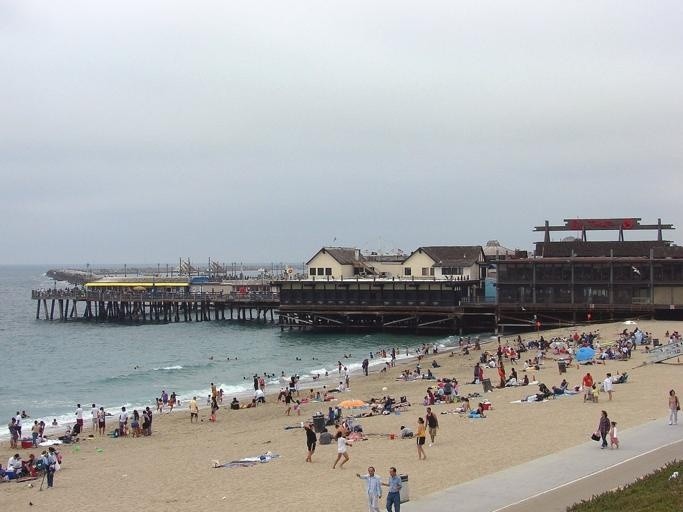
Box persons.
[355,466,383,511]
[362,327,680,461]
[45,447,60,487]
[155,383,223,422]
[230,370,301,415]
[0,453,35,477]
[294,352,365,469]
[32,284,276,304]
[37,450,50,490]
[9,403,153,450]
[379,467,402,512]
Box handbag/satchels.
[592,432,600,442]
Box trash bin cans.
[558,362,566,375]
[482,378,492,393]
[653,339,659,347]
[312,415,325,433]
